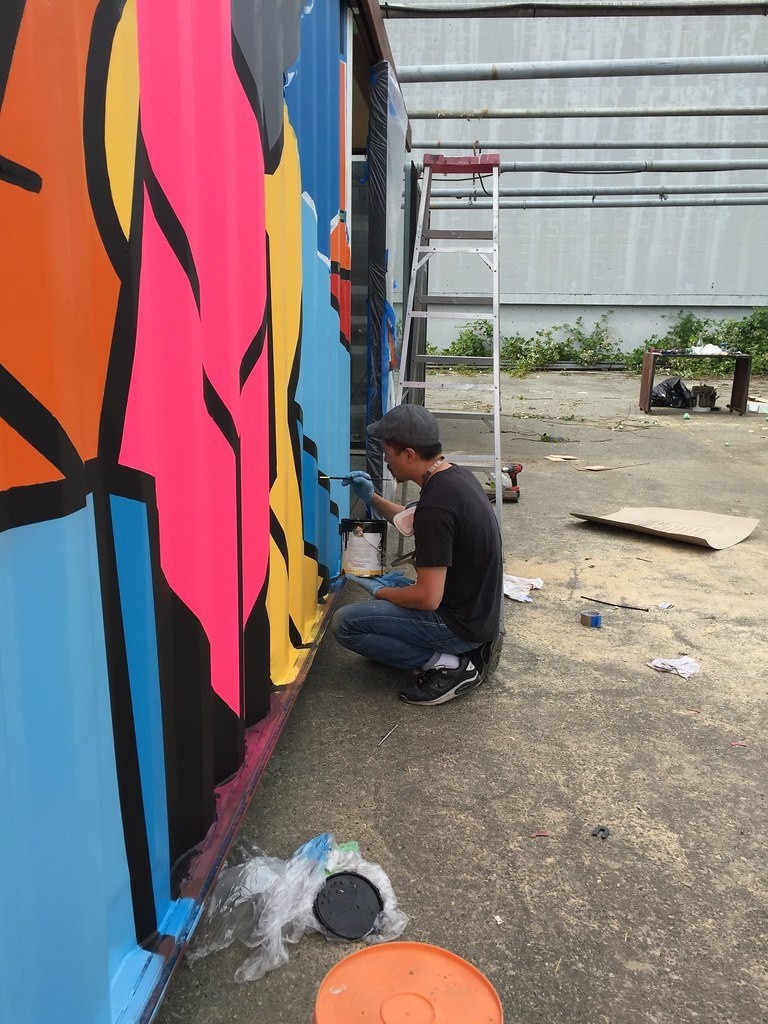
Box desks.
[638,351,754,416]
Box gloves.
[344,573,384,599]
[341,471,374,504]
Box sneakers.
[471,632,504,683]
[396,653,482,706]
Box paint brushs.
[318,475,392,481]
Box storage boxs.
[747,396,768,414]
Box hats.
[364,405,439,447]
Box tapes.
[580,610,602,628]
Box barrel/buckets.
[340,518,387,577]
[692,385,714,412]
[313,941,504,1024]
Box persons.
[333,403,507,706]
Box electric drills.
[501,463,523,497]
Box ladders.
[396,153,503,558]
